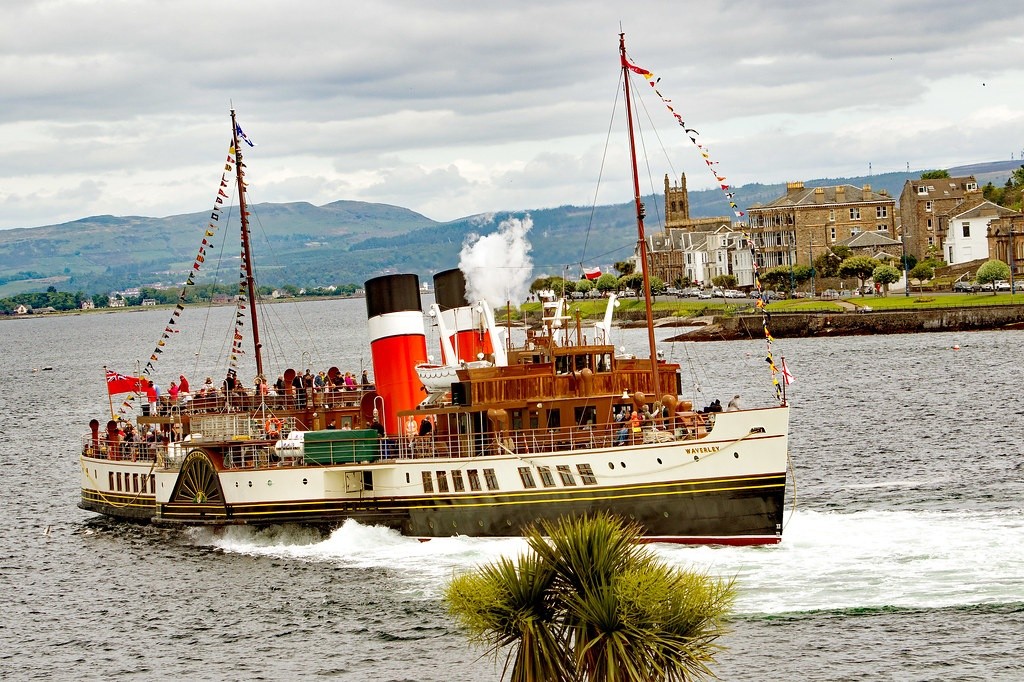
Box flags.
[104,367,150,395]
[619,47,795,408]
[113,121,255,421]
[583,267,601,279]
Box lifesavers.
[265,418,281,435]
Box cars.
[571,286,873,300]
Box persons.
[907,280,913,291]
[966,285,977,296]
[118,369,385,457]
[613,395,741,447]
[762,294,770,304]
[405,415,432,448]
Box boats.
[69,20,792,546]
